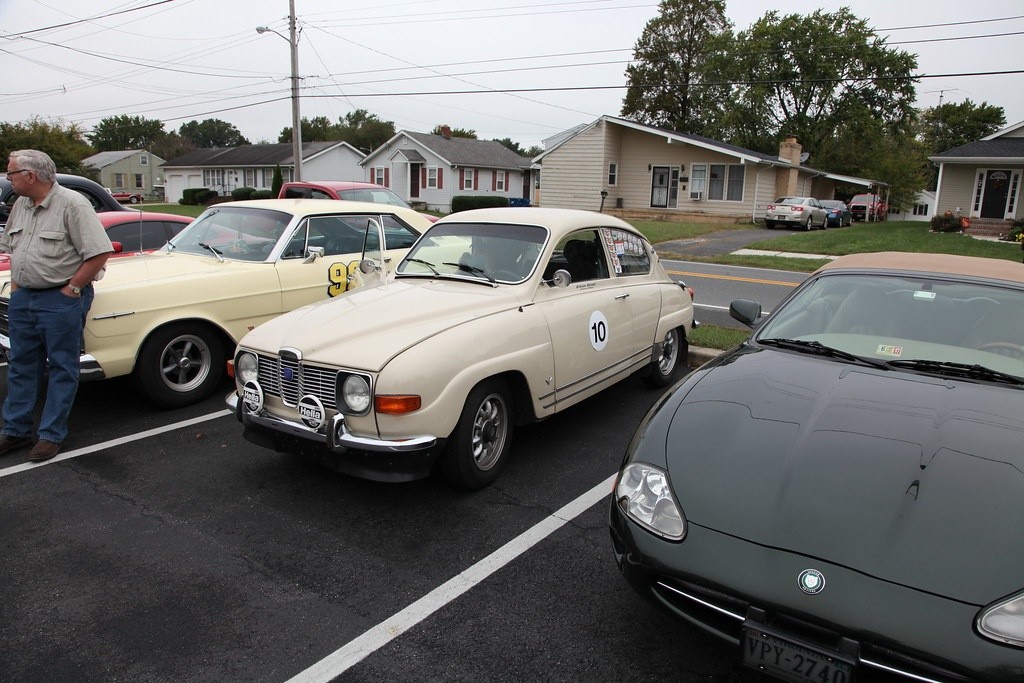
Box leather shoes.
[28,439,62,462]
[0,433,32,456]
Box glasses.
[6,170,31,178]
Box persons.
[0,149,115,462]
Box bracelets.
[69,284,81,294]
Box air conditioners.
[690,191,703,200]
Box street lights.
[256,0,302,181]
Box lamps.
[648,164,651,170]
[681,164,684,171]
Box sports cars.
[608,252,1024,683]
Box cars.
[765,195,888,231]
[0,173,694,488]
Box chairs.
[562,239,598,282]
